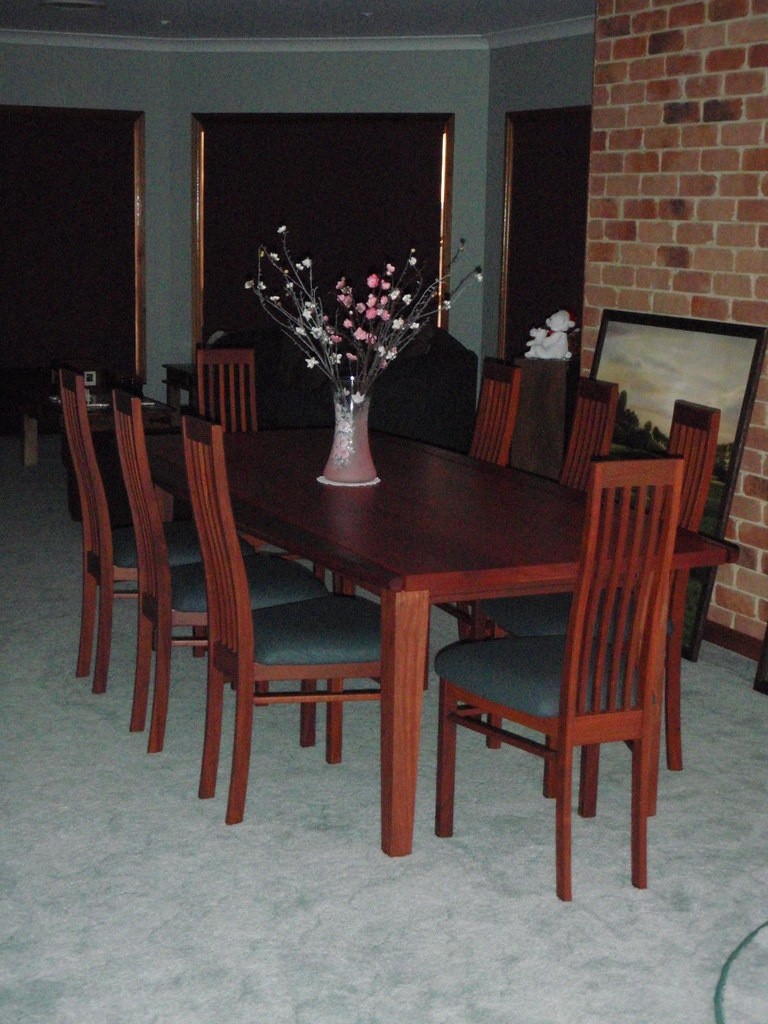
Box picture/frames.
[590,308,768,666]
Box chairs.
[111,386,327,755]
[474,400,721,771]
[56,366,254,695]
[193,347,259,433]
[434,454,689,905]
[179,409,383,827]
[456,352,622,639]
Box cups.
[85,388,97,405]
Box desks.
[12,382,177,468]
[140,419,742,853]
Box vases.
[317,377,383,487]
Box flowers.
[242,223,482,403]
